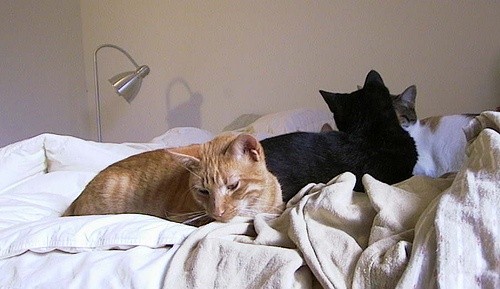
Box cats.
[356,82,480,178]
[259,69,417,208]
[60,133,282,229]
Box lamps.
[92,44,151,142]
